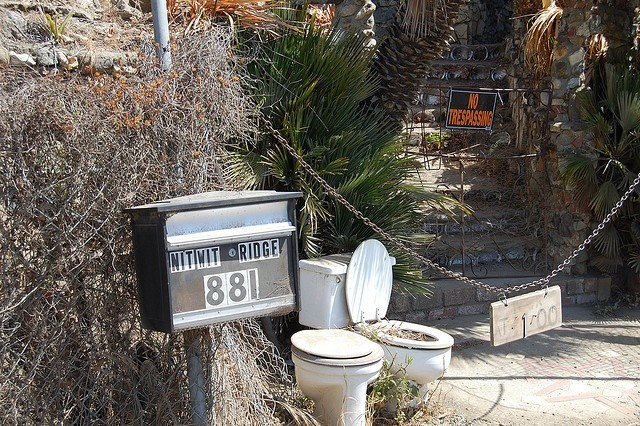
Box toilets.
[291,329,384,425]
[299,239,454,414]
